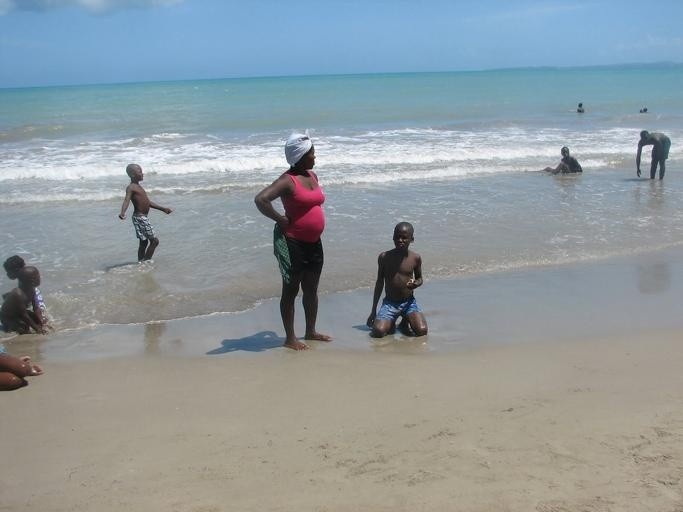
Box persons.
[541,147,583,174]
[361,222,429,339]
[0,265,47,335]
[2,255,52,322]
[635,131,672,180]
[253,133,334,352]
[117,162,173,260]
[0,350,43,390]
